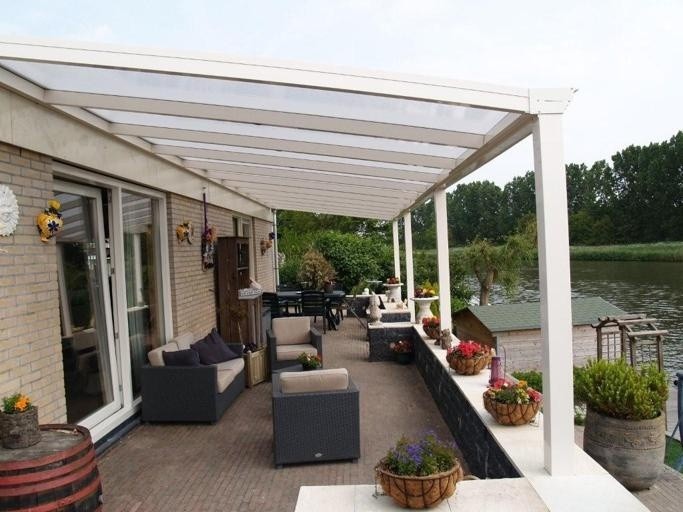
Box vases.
[396,353,412,364]
[424,326,440,339]
[446,353,493,376]
[375,462,462,510]
[1,407,42,448]
[482,393,540,426]
[410,295,439,323]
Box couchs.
[139,338,246,421]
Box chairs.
[266,315,360,469]
[261,283,343,334]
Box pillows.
[163,328,240,367]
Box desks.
[275,291,345,329]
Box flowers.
[389,339,414,353]
[446,339,489,358]
[2,394,32,414]
[414,286,434,297]
[486,379,543,406]
[422,317,440,327]
[371,429,462,476]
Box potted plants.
[571,353,670,492]
[383,277,405,303]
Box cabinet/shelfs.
[217,236,249,344]
